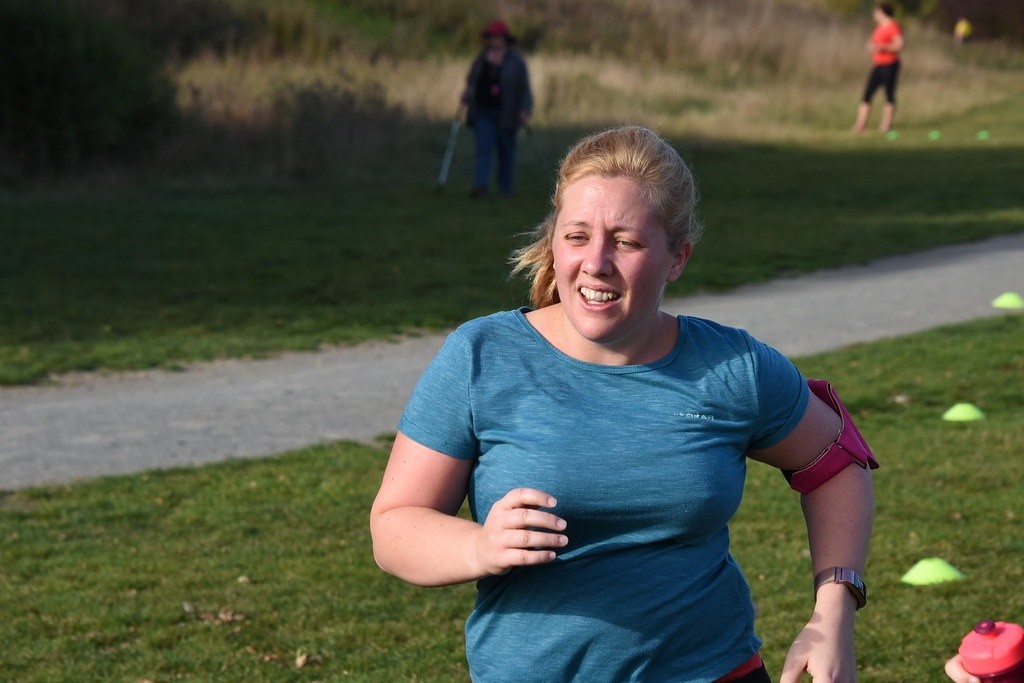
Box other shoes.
[469,185,487,198]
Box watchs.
[814,567,867,609]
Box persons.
[954,17,973,45]
[848,2,904,131]
[368,125,874,683]
[459,21,533,201]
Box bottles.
[958,619,1024,683]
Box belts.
[717,651,762,683]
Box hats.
[481,20,508,38]
